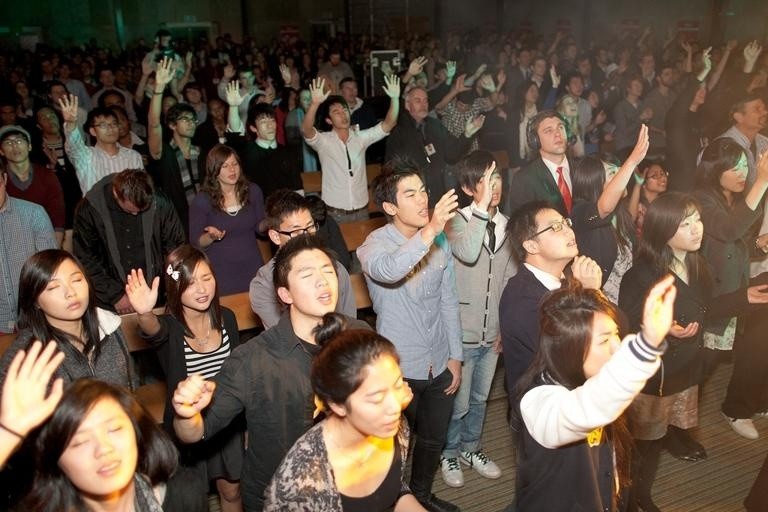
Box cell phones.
[443,167,460,212]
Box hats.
[1,124,31,145]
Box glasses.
[93,121,123,129]
[275,222,319,238]
[175,116,199,123]
[527,218,572,240]
[646,170,670,179]
[3,136,26,145]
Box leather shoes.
[665,433,708,462]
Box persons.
[1,16,767,511]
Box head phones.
[526,111,578,149]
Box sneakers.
[720,409,760,440]
[439,455,464,488]
[459,450,502,479]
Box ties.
[486,221,496,253]
[556,168,572,217]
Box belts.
[326,202,369,215]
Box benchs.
[0,148,515,425]
